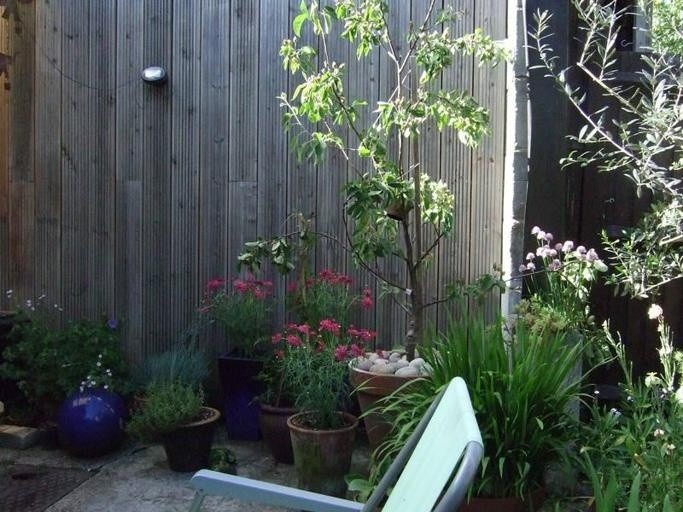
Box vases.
[257,397,303,465]
[217,351,267,442]
[55,388,128,456]
[514,334,584,423]
[286,412,358,500]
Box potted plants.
[36,334,92,418]
[132,330,214,414]
[349,293,619,512]
[276,0,516,449]
[126,375,220,473]
[0,323,48,410]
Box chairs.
[186,377,484,512]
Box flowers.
[258,320,383,430]
[67,311,121,363]
[79,349,136,394]
[195,271,279,359]
[518,225,608,330]
[285,270,374,345]
[247,323,318,407]
[6,289,62,338]
